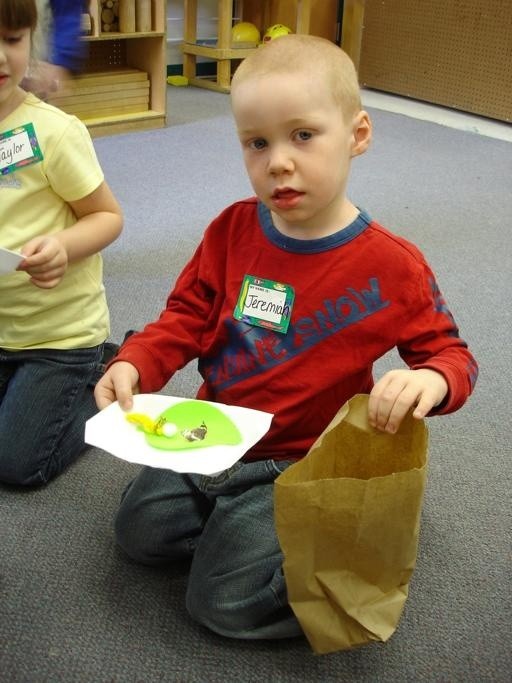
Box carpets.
[0,78,512,681]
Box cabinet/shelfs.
[45,0,168,136]
[180,0,310,94]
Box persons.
[91,32,481,646]
[0,0,141,493]
